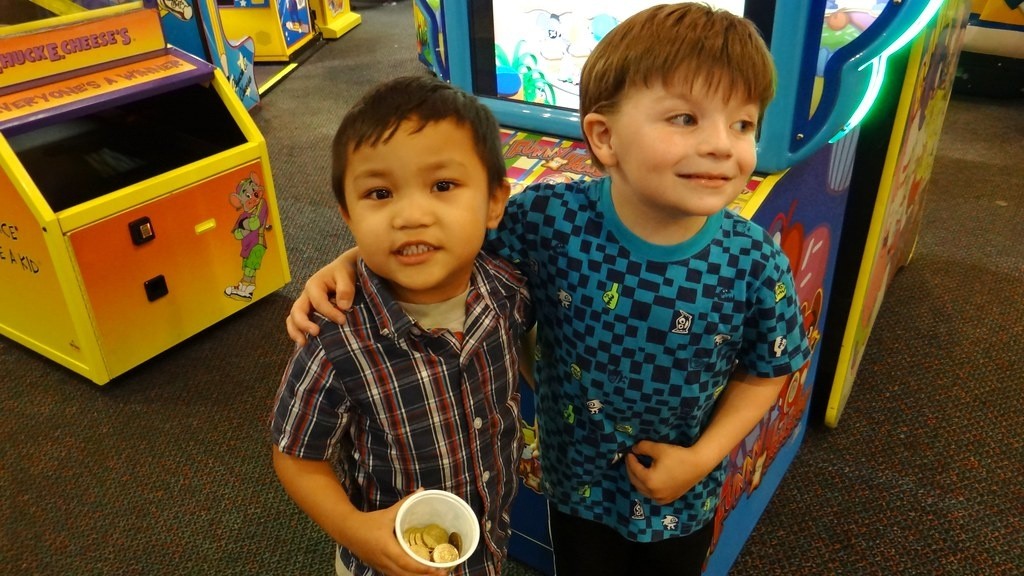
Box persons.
[262,73,538,576]
[285,2,814,576]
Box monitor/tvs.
[470,0,774,113]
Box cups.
[395,490,480,576]
